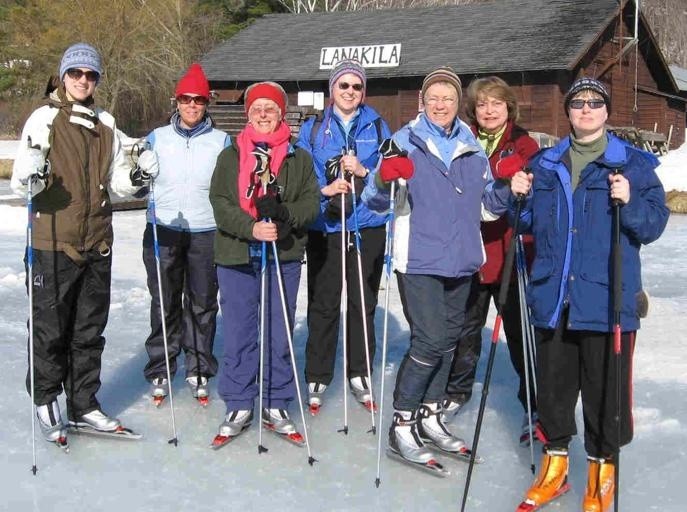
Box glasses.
[339,82,363,91]
[569,99,606,109]
[423,96,459,105]
[67,68,99,81]
[250,109,278,114]
[177,95,208,105]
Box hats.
[564,77,611,119]
[60,43,101,83]
[176,64,209,100]
[329,59,366,98]
[244,81,288,119]
[422,64,462,104]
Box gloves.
[274,218,291,240]
[255,196,289,221]
[381,157,413,182]
[496,151,526,179]
[129,151,159,197]
[17,136,52,185]
[131,139,150,163]
[325,174,365,221]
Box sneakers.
[261,408,296,433]
[351,375,376,405]
[186,376,209,398]
[67,408,121,431]
[583,456,616,512]
[518,411,537,446]
[416,402,465,452]
[219,404,254,436]
[35,399,66,442]
[442,396,469,423]
[388,409,434,463]
[149,377,172,397]
[306,381,328,406]
[526,446,570,504]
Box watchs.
[365,166,370,177]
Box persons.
[143,63,232,405]
[511,76,671,511]
[437,76,544,446]
[10,43,159,454]
[360,67,525,475]
[209,82,319,450]
[295,60,392,415]
